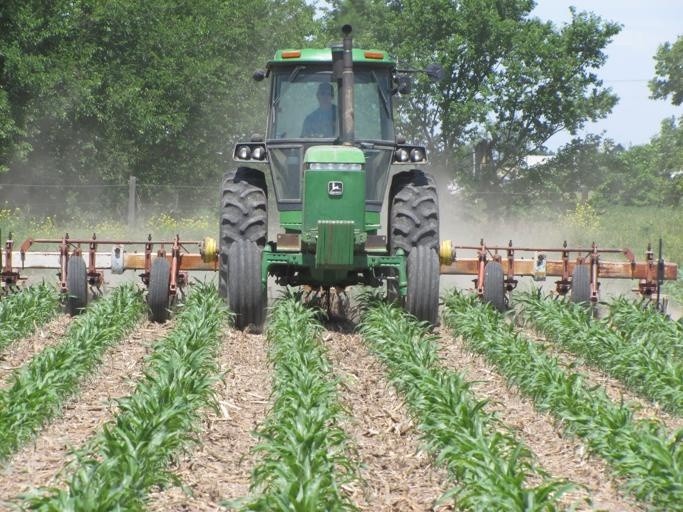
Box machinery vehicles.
[0,23,678,335]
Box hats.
[318,82,334,95]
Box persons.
[299,82,337,139]
